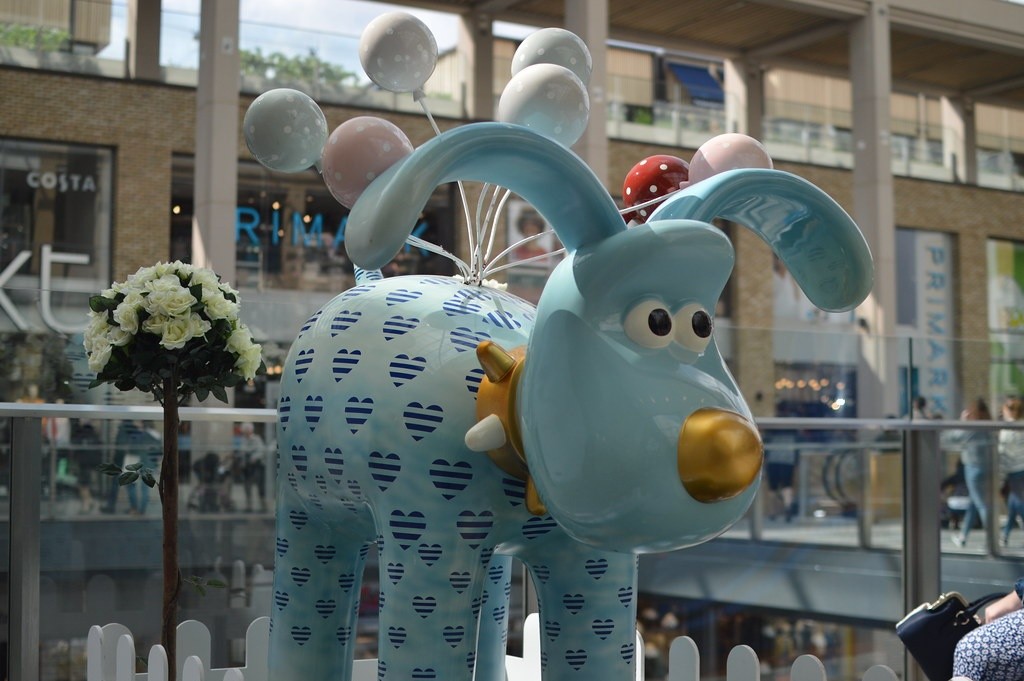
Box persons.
[100,421,160,514]
[997,400,1024,546]
[70,425,94,512]
[876,416,900,454]
[939,464,967,528]
[236,423,267,513]
[944,398,992,545]
[953,577,1024,681]
[902,396,928,420]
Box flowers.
[83,258,269,681]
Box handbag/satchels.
[895,591,1010,680]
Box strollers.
[185,452,245,515]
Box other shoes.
[951,536,965,547]
[999,538,1009,548]
[100,506,114,514]
